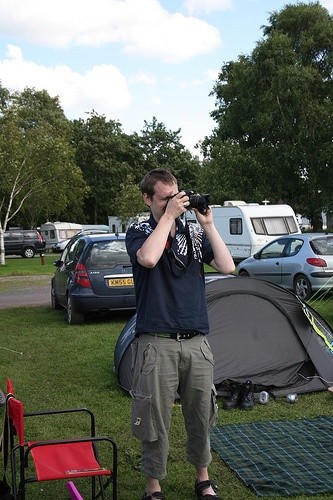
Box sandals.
[194,478,221,500]
[142,491,166,500]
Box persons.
[125,168,236,500]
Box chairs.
[2,378,118,500]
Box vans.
[210,200,304,261]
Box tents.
[113,273,333,405]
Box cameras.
[176,190,210,215]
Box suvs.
[3,227,45,258]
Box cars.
[235,233,333,300]
[49,229,137,326]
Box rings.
[184,203,186,208]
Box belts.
[147,331,201,341]
